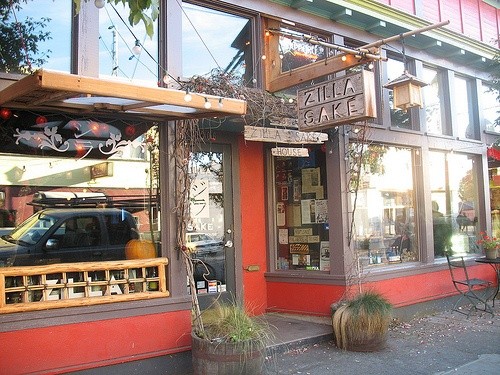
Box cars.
[0,208,141,269]
[184,230,220,257]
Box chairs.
[79,223,98,246]
[60,218,81,247]
[446,255,496,320]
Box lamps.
[184,88,192,102]
[204,97,211,109]
[382,38,430,114]
[22,166,26,172]
[94,0,105,9]
[132,39,142,55]
[218,99,224,108]
[49,162,53,168]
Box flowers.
[476,231,500,249]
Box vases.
[486,248,497,259]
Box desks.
[474,257,500,307]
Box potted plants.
[190,289,289,375]
[331,285,393,352]
